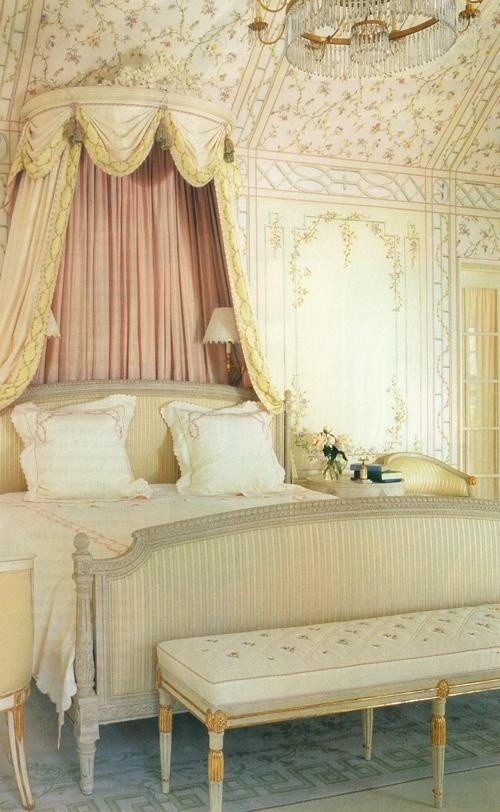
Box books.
[351,464,389,472]
[353,471,404,482]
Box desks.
[306,475,405,498]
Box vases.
[323,455,342,481]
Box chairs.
[0,553,38,811]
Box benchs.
[157,603,500,812]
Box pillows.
[11,394,153,503]
[160,400,285,496]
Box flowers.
[312,426,349,461]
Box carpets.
[0,679,499,812]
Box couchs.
[383,452,478,497]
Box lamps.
[248,0,482,79]
[202,307,240,385]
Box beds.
[0,379,499,796]
